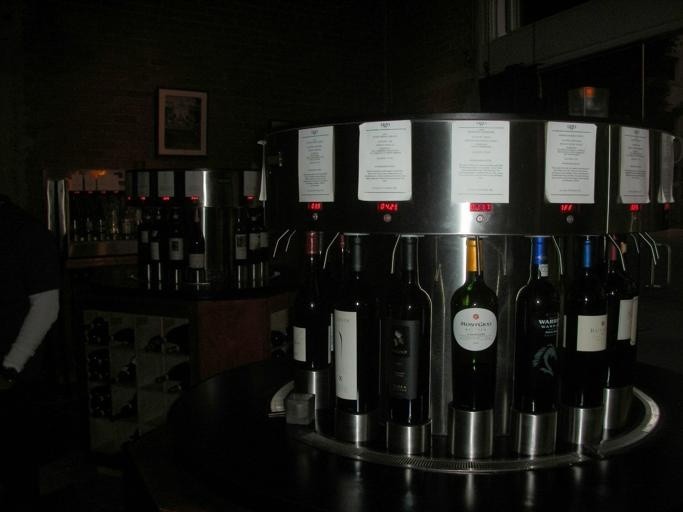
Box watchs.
[1,366,18,385]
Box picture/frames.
[155,87,207,157]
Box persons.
[0,194,64,512]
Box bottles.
[513,234,561,412]
[449,234,498,412]
[603,236,641,385]
[83,323,197,442]
[332,237,380,415]
[378,236,433,426]
[293,228,330,370]
[70,203,136,239]
[566,233,608,406]
[139,203,205,272]
[234,213,269,260]
